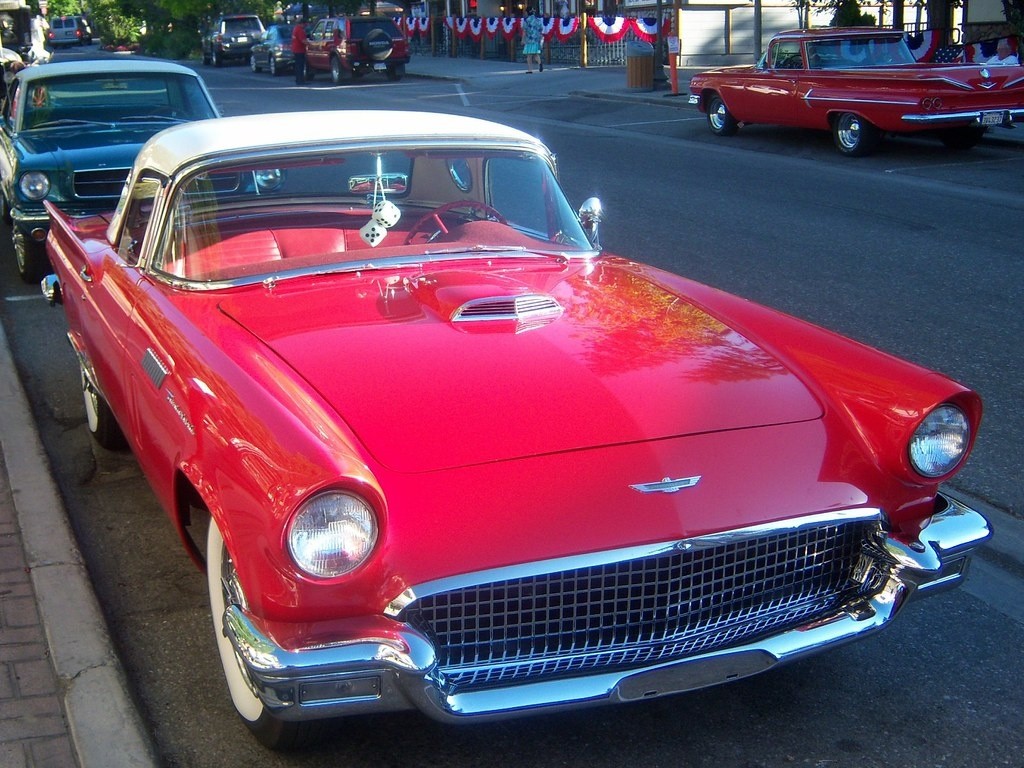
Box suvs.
[304,16,411,85]
[202,14,266,67]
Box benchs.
[22,103,176,130]
[161,204,471,276]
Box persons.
[292,18,310,85]
[521,7,544,74]
[987,38,1019,65]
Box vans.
[49,16,93,49]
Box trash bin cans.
[626,40,654,92]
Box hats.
[297,18,310,24]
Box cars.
[249,23,312,76]
[688,26,1024,156]
[0,60,224,285]
[42,112,992,754]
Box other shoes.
[539,63,543,72]
[525,72,533,74]
